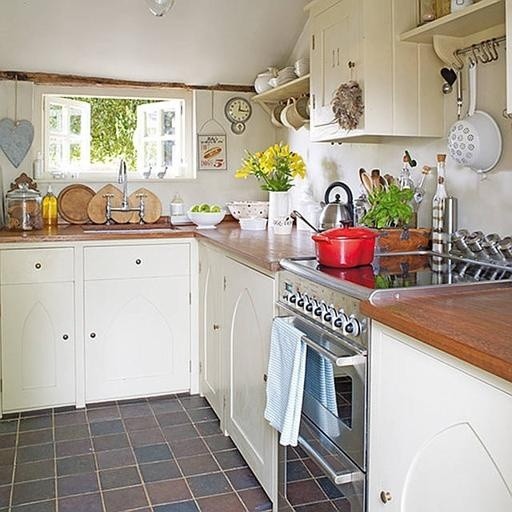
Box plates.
[276,66,296,85]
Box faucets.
[118,158,128,207]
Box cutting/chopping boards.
[56,184,162,225]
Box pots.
[254,66,276,94]
[311,220,379,268]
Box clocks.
[224,96,252,134]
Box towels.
[264,318,308,448]
[295,317,341,441]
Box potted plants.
[361,183,431,250]
[372,253,428,292]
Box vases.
[268,190,294,236]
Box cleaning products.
[171,193,183,216]
[41,185,58,227]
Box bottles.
[6,183,41,231]
[169,192,184,215]
[432,244,445,288]
[431,154,447,243]
[40,185,58,225]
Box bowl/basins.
[187,210,225,229]
[225,201,269,224]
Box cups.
[273,216,294,235]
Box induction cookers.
[278,250,512,307]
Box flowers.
[233,141,307,192]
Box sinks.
[79,220,177,236]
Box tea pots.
[319,181,354,231]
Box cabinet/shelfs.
[364,317,512,512]
[78,239,196,407]
[197,236,278,505]
[1,242,78,414]
[303,0,445,141]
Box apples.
[193,204,221,212]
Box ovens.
[273,268,368,512]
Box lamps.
[145,0,174,18]
